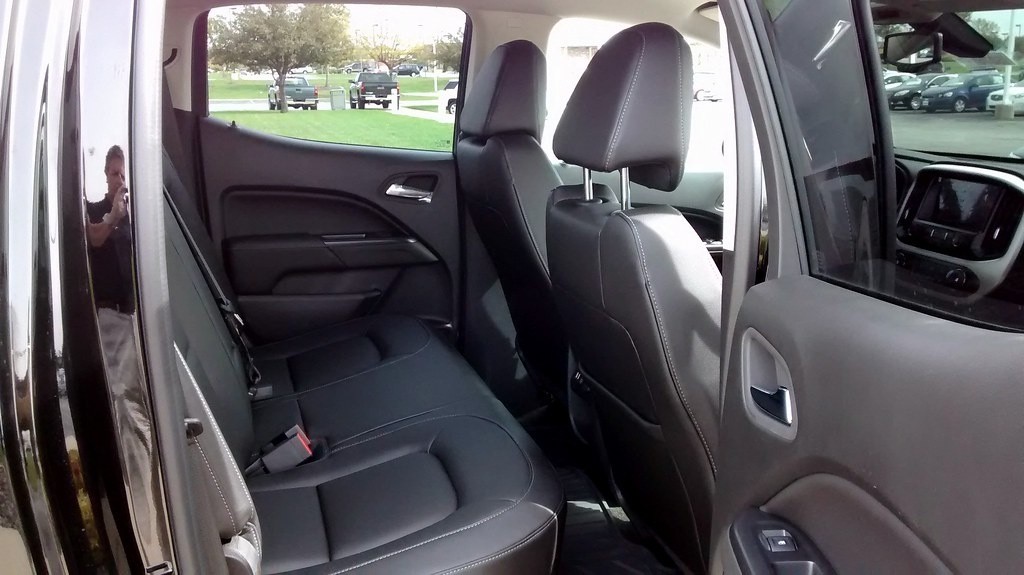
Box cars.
[287,64,314,74]
[691,70,723,102]
[440,79,458,115]
[1,1,1024,574]
[881,45,1024,116]
[391,63,422,78]
[343,61,373,75]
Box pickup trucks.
[267,75,320,110]
[348,71,400,110]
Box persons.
[83,145,170,564]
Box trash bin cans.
[330,89,346,111]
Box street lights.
[356,29,361,45]
[372,23,378,46]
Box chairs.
[159,21,725,575]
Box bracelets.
[102,212,120,231]
